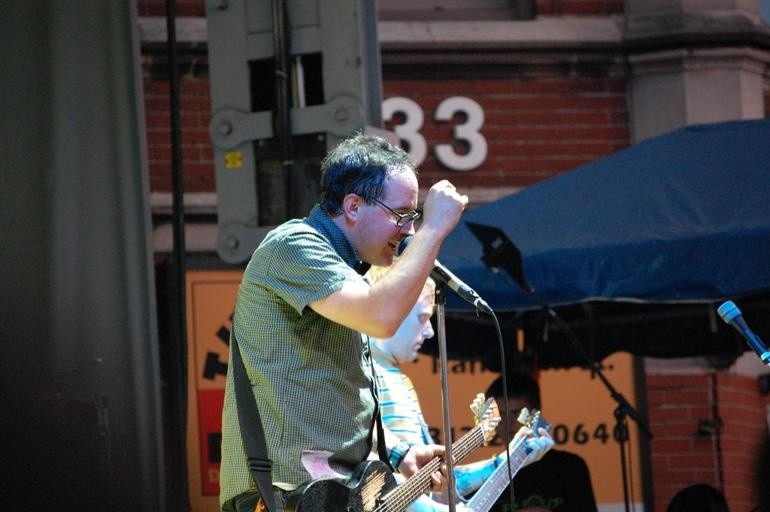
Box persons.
[461,373,598,512]
[217,132,469,511]
[361,262,555,510]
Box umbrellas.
[392,116,770,511]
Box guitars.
[422,407,552,512]
[225,393,502,512]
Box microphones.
[392,235,495,317]
[715,299,770,367]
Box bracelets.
[491,454,500,469]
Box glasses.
[364,193,421,227]
